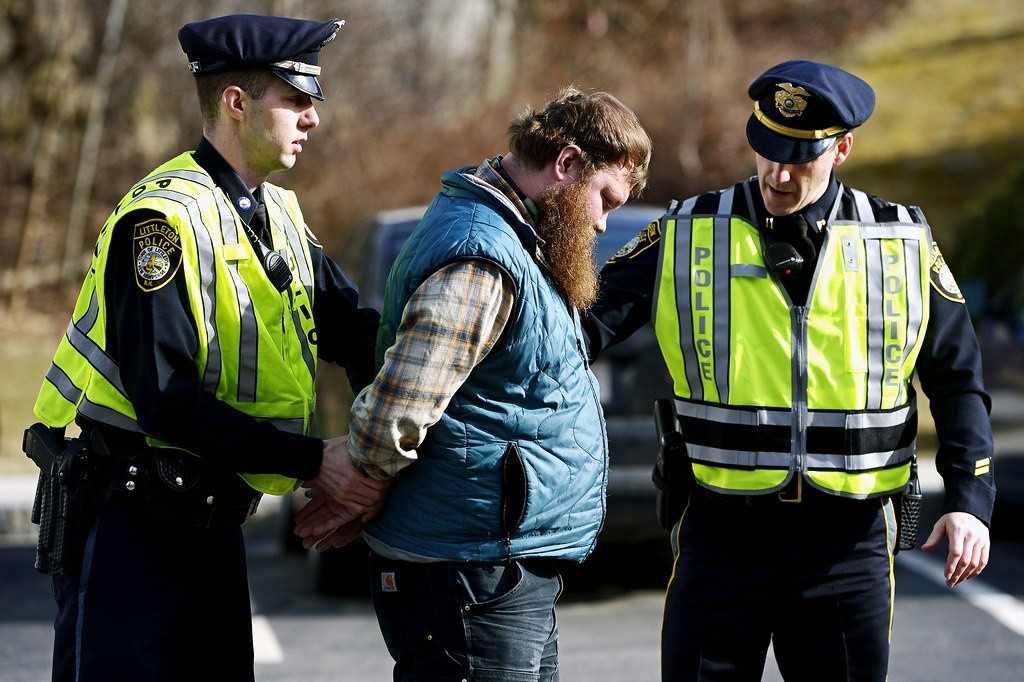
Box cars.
[276,203,670,597]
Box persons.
[25,15,384,681]
[294,86,651,681]
[580,59,995,682]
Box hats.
[746,59,877,164]
[178,13,346,102]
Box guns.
[650,396,684,532]
[17,421,97,587]
